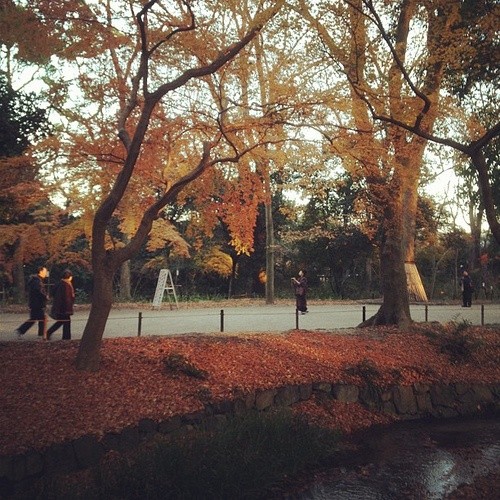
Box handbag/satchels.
[295,286,303,296]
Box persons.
[290,270,308,315]
[44,269,74,341]
[14,266,50,340]
[459,271,472,308]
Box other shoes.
[301,310,309,315]
[14,330,25,340]
[36,336,46,340]
[45,332,51,341]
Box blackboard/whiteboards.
[152,269,168,307]
